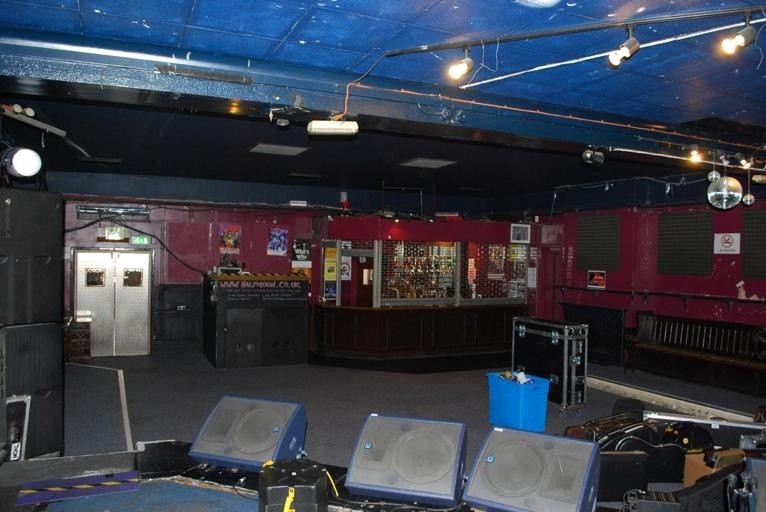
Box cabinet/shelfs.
[218,302,309,368]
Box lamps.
[723,12,757,56]
[609,25,641,68]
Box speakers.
[0,322,66,462]
[344,414,467,507]
[188,393,308,473]
[461,425,602,512]
[0,188,66,326]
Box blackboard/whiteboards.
[217,278,308,300]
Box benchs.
[626,310,766,402]
